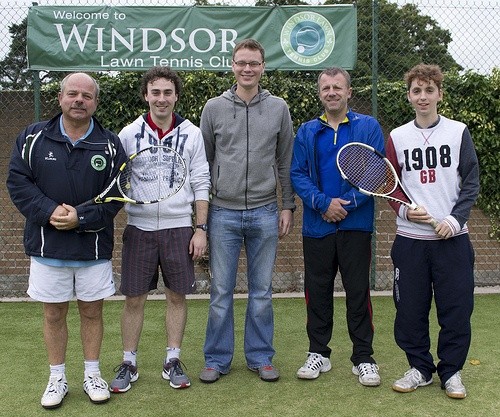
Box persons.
[290,67,387,387]
[6,73,128,410]
[198,38,296,383]
[109,66,211,393]
[384,64,479,399]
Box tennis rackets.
[336,141,439,228]
[75,146,187,209]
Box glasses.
[233,59,264,69]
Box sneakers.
[109,361,138,392]
[41,374,69,409]
[163,358,190,388]
[198,368,220,381]
[352,362,381,385]
[83,371,110,403]
[247,365,280,382]
[296,352,331,378]
[443,370,467,398]
[393,367,433,392]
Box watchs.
[196,224,207,231]
